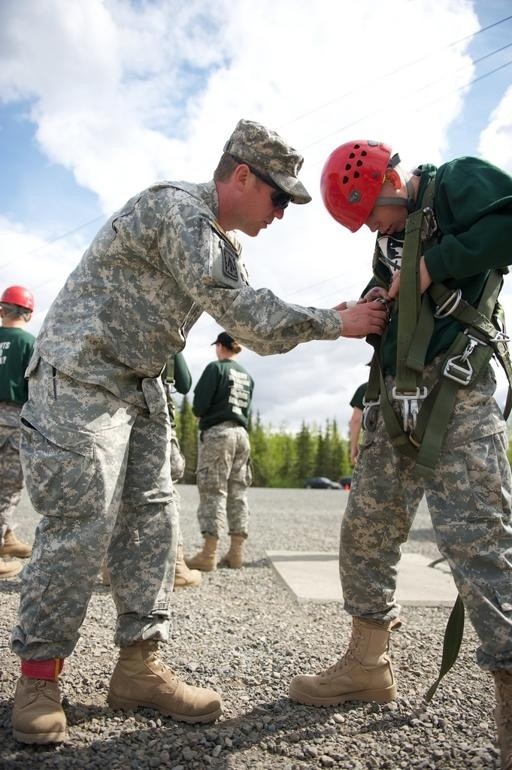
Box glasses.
[233,155,294,210]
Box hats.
[210,332,239,349]
[220,117,313,206]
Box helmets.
[319,138,393,235]
[0,285,35,313]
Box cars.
[304,477,344,489]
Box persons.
[10,120,390,746]
[289,139,512,770]
[102,353,202,589]
[184,332,254,570]
[350,382,369,464]
[0,286,40,579]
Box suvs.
[338,478,353,490]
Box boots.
[10,656,68,747]
[0,530,35,580]
[221,534,248,570]
[287,615,399,708]
[171,544,202,588]
[183,533,220,574]
[491,667,512,770]
[105,640,225,725]
